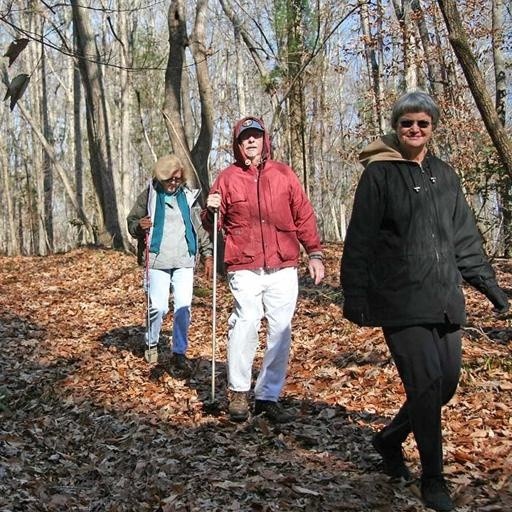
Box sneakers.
[228,391,250,421]
[144,345,158,364]
[372,430,411,480]
[173,354,192,371]
[255,400,291,424]
[419,475,454,511]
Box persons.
[126,154,212,369]
[199,116,327,424]
[340,92,511,512]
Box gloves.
[138,218,153,230]
[342,296,369,325]
[204,255,215,281]
[484,285,510,314]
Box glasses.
[162,177,182,183]
[396,120,432,129]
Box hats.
[235,118,265,138]
